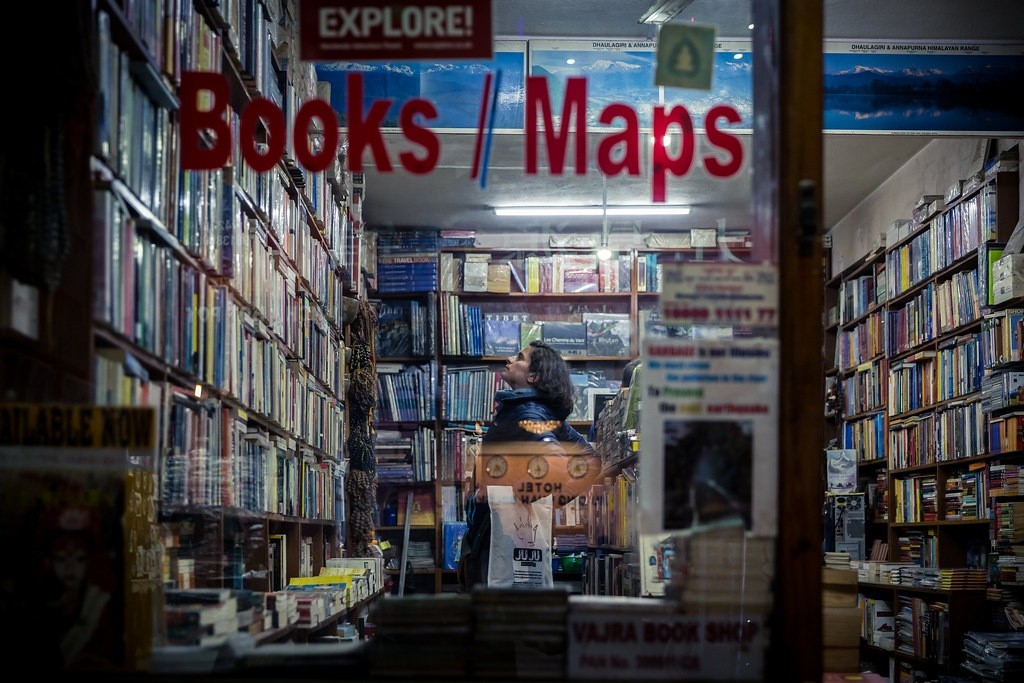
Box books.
[1,0,1024,681]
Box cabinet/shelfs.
[0,0,1024,682]
